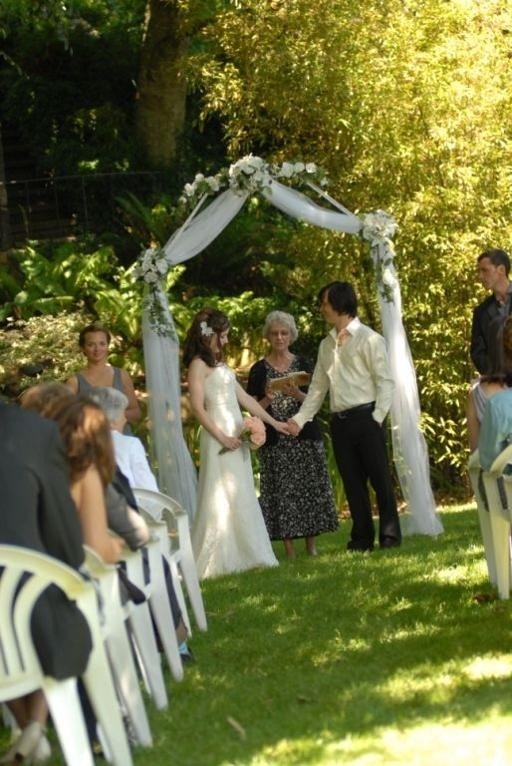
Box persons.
[286,280,403,550]
[182,309,291,580]
[248,311,339,558]
[471,249,512,376]
[465,315,512,509]
[63,325,142,438]
[0,382,193,764]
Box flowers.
[361,209,399,302]
[134,246,176,342]
[177,152,316,206]
[219,417,266,456]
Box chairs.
[468,443,512,601]
[0,487,208,766]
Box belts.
[332,402,375,418]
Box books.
[268,371,310,393]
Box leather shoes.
[346,535,401,552]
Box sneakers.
[0,721,52,765]
[181,646,197,662]
